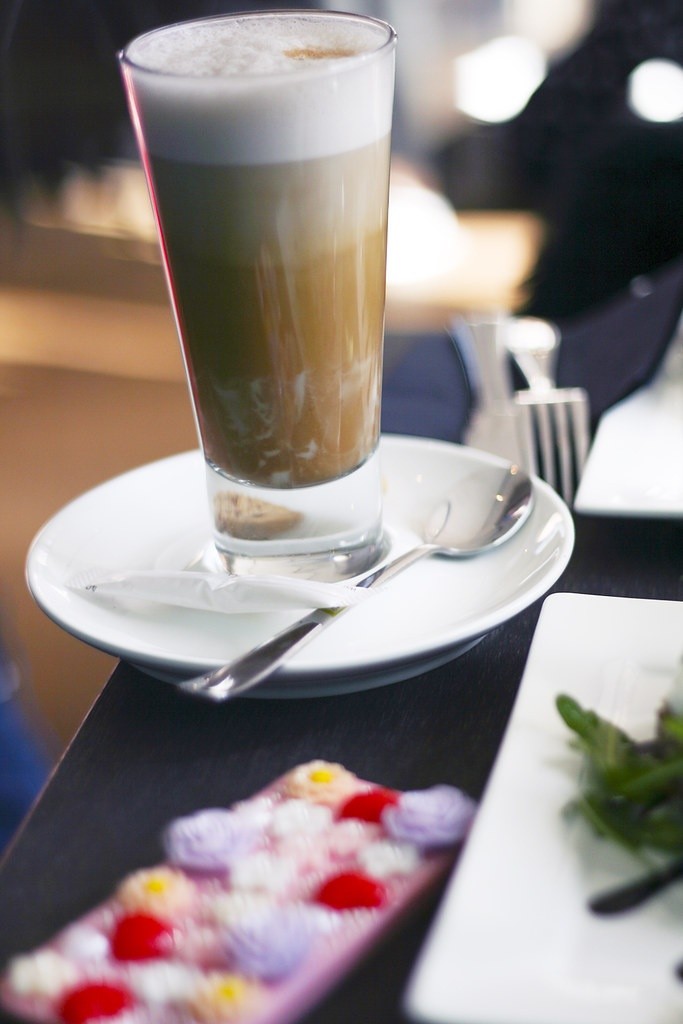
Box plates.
[576,329,682,519]
[25,433,575,700]
[396,592,683,1024]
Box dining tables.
[0,256,682,1024]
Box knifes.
[447,319,522,469]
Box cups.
[117,8,398,582]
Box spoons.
[180,465,534,700]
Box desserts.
[2,760,477,1024]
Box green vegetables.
[556,693,683,861]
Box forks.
[500,315,591,508]
